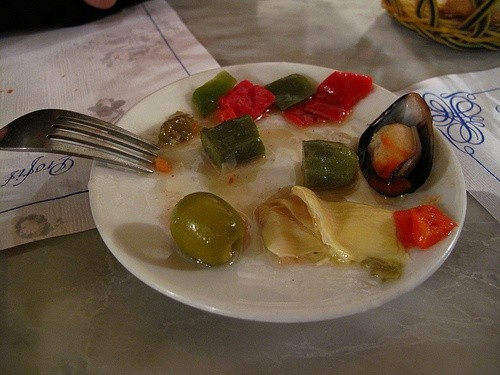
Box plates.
[88,61,468,323]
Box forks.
[0,109,160,173]
